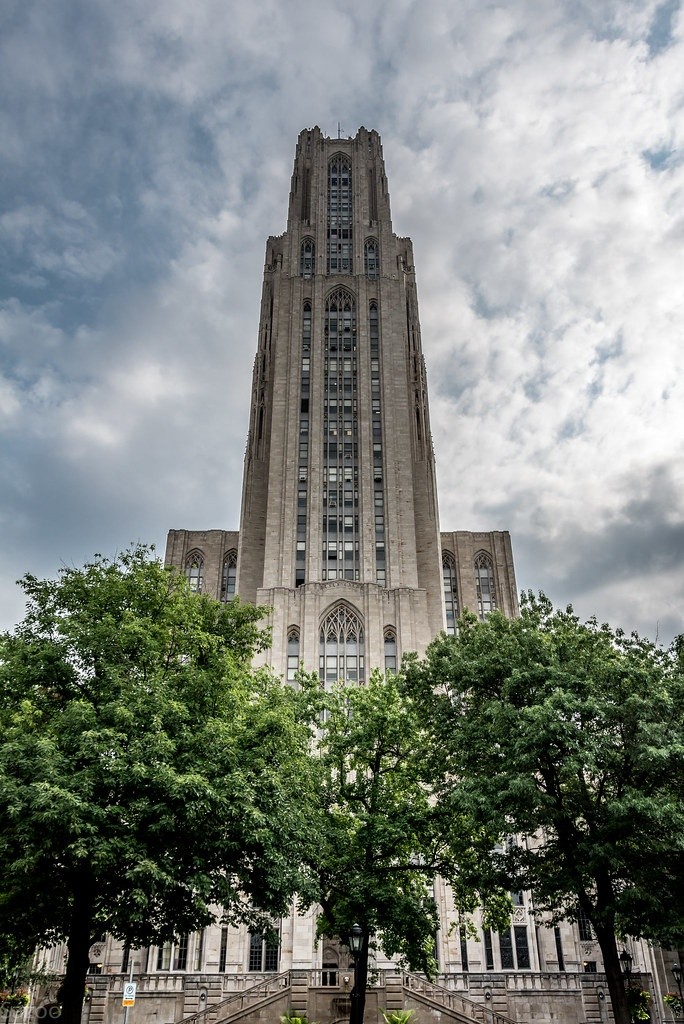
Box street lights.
[670,963,684,1015]
[619,950,638,1024]
[5,970,19,1024]
